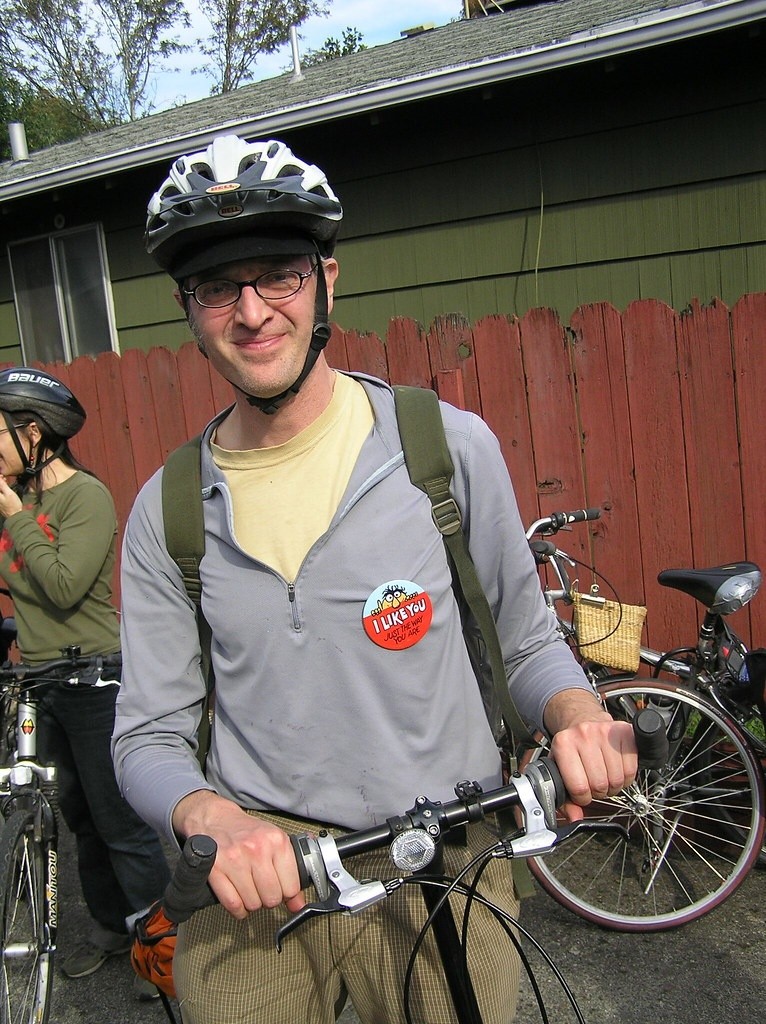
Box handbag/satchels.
[130,898,178,998]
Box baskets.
[573,592,647,674]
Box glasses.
[182,264,318,309]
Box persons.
[0,367,161,1000]
[110,134,638,1024]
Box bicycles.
[499,507,766,936]
[0,615,124,1024]
[125,707,671,1024]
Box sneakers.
[59,925,132,978]
[133,973,163,1000]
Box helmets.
[142,134,343,284]
[0,367,86,439]
